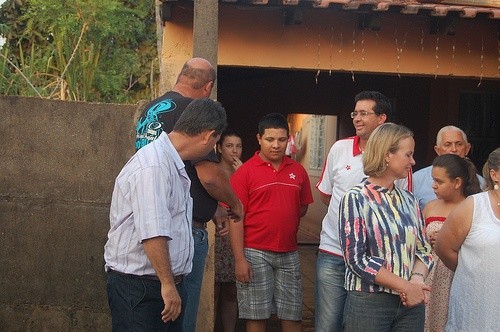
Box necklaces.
[493,190,500,207]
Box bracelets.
[410,273,426,283]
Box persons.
[432,147,500,332]
[217,112,315,332]
[420,152,485,332]
[411,124,487,215]
[337,121,437,332]
[103,97,229,332]
[134,57,245,332]
[315,89,414,332]
[213,127,245,331]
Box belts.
[190,220,208,229]
[108,269,183,285]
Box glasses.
[350,111,377,118]
